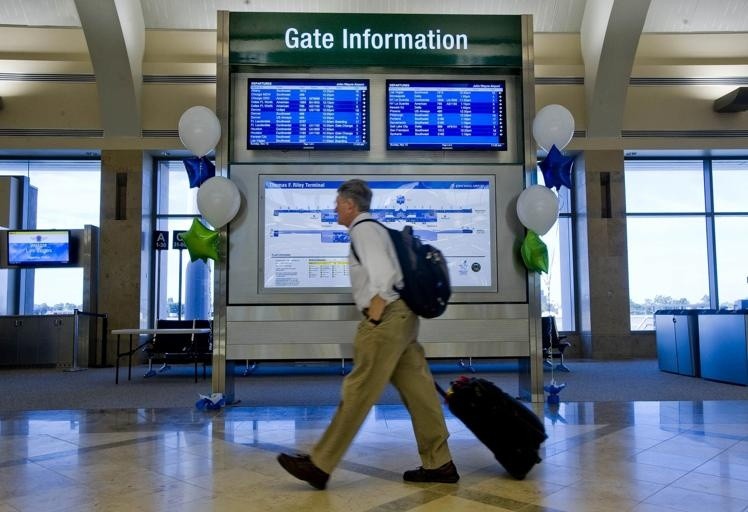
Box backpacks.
[351,218,451,319]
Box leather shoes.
[403,463,460,483]
[275,452,330,490]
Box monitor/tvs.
[246,78,370,151]
[385,79,507,152]
[7,230,72,265]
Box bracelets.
[364,313,383,326]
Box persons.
[276,180,460,490]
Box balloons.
[531,103,575,153]
[197,175,241,230]
[538,144,575,189]
[177,105,222,159]
[515,183,560,238]
[180,218,221,263]
[181,156,216,188]
[520,231,549,276]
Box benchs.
[541,316,572,372]
[143,319,213,384]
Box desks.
[111,328,211,384]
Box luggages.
[435,375,548,481]
